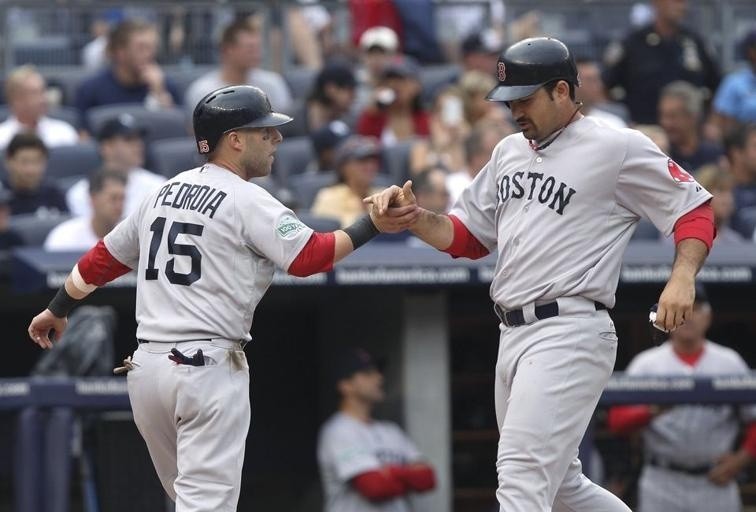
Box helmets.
[484,37,579,109]
[192,84,293,155]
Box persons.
[320,343,439,511]
[601,281,756,512]
[0,1,756,251]
[360,33,718,512]
[21,83,421,512]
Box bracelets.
[47,281,86,318]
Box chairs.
[0,0,756,270]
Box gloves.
[169,348,204,366]
[112,356,137,373]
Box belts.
[501,302,605,328]
